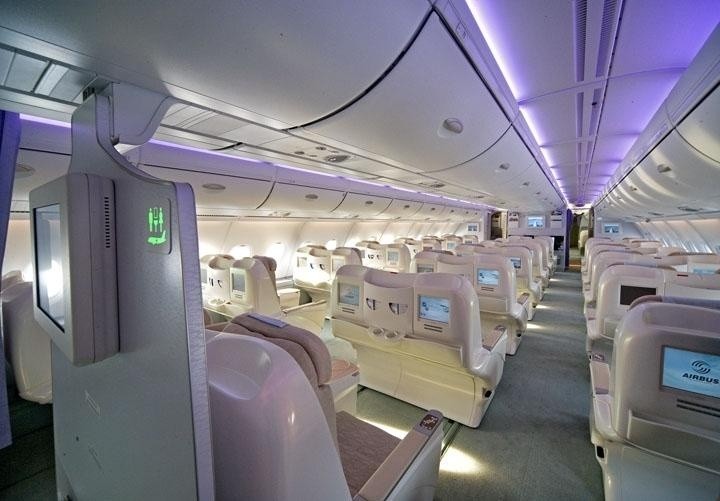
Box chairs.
[200,235,555,429]
[581,237,720,501]
[200,313,444,500]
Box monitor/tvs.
[467,223,479,233]
[693,262,720,274]
[338,283,359,306]
[526,215,546,228]
[28,173,119,368]
[620,285,656,306]
[297,240,534,286]
[200,269,245,292]
[419,295,449,325]
[602,223,622,236]
[660,345,720,400]
